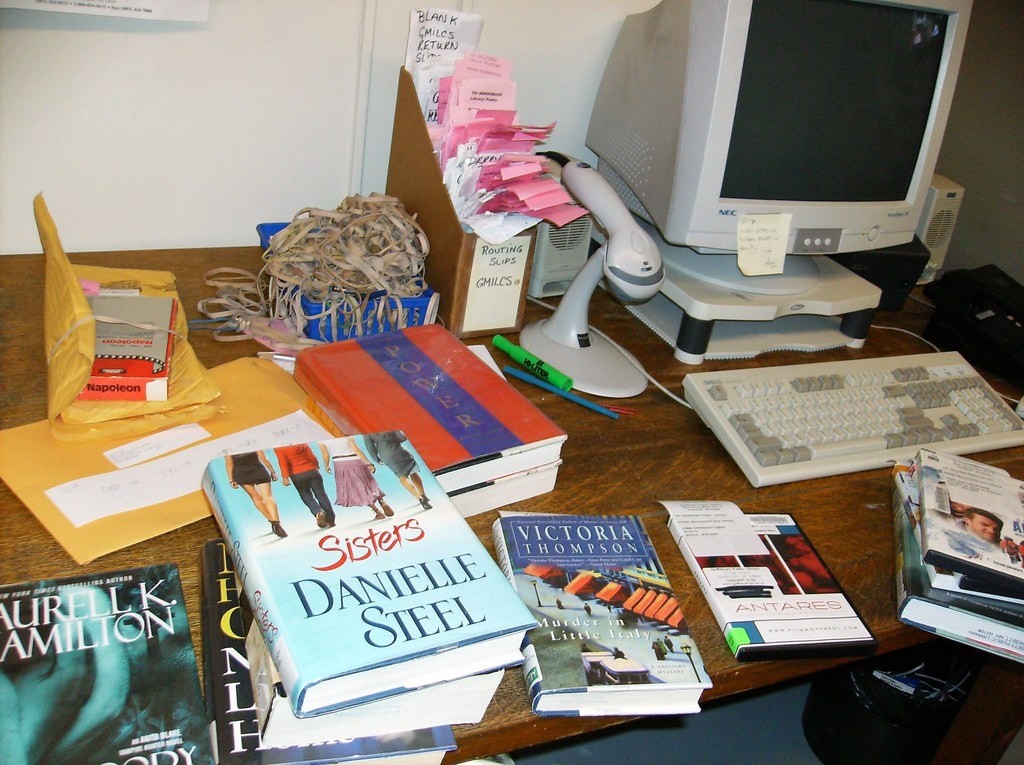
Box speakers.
[529,209,594,299]
[915,173,964,284]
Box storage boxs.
[260,222,434,342]
[385,65,539,341]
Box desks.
[0,247,1024,765]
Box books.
[891,463,1024,606]
[915,447,1024,591]
[893,488,1024,665]
[1,324,878,765]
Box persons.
[950,500,1024,567]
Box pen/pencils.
[503,364,621,419]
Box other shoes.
[382,502,394,517]
[419,494,433,510]
[376,513,386,519]
[328,520,335,527]
[317,512,327,528]
[272,522,287,537]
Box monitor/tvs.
[584,0,975,296]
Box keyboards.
[682,350,1024,489]
